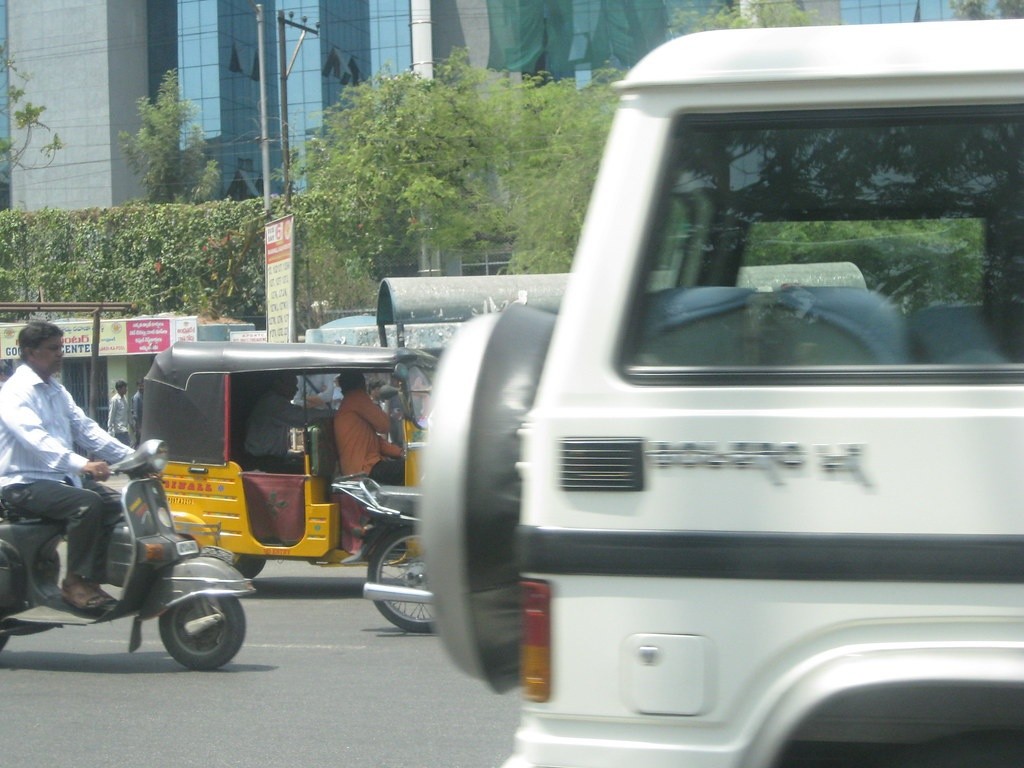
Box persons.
[105,380,147,448]
[0,319,163,609]
[244,372,403,545]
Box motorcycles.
[330,472,439,632]
[0,321,257,670]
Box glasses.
[31,344,64,352]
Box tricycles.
[138,340,438,579]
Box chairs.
[635,285,908,366]
[916,304,1022,364]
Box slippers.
[62,578,118,611]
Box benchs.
[306,418,385,492]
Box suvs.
[418,19,1024,768]
